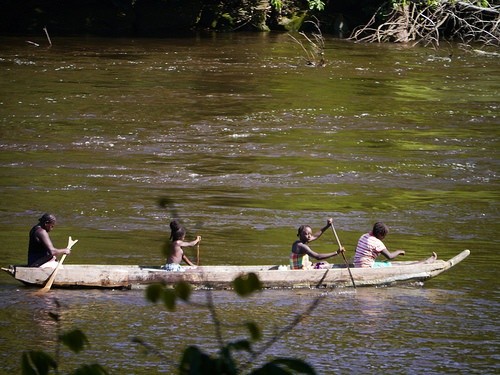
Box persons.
[166,221,201,271]
[28,212,71,268]
[290,218,346,270]
[352,221,437,268]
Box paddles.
[330,221,357,289]
[196,235,200,266]
[41,235,79,292]
